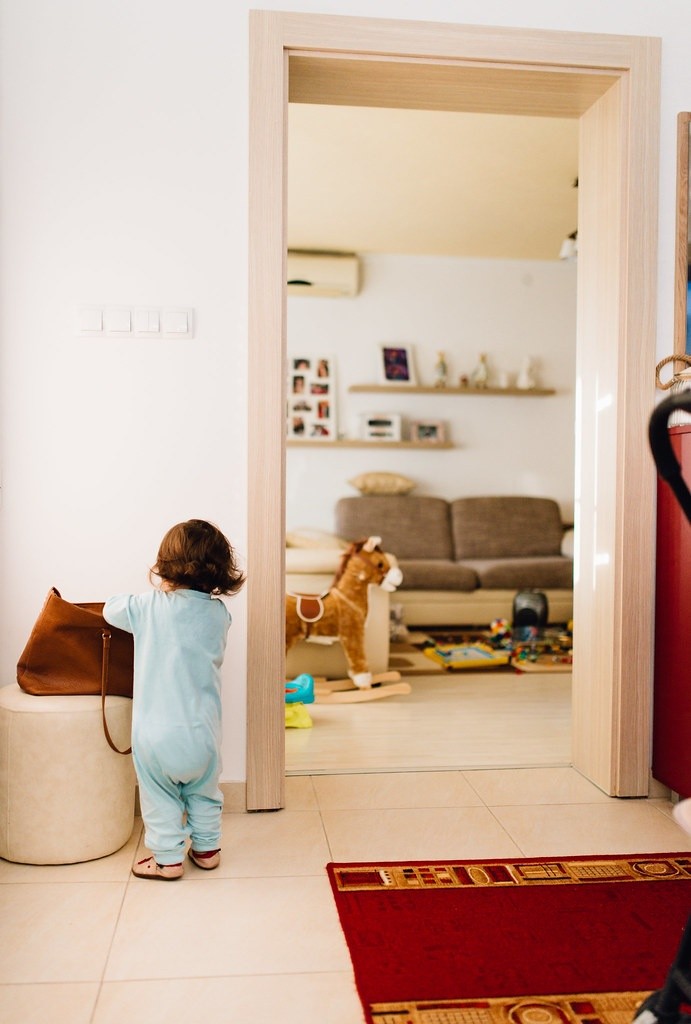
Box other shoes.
[132,856,191,878]
[189,845,220,868]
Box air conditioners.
[287,243,359,298]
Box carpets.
[325,852,691,1024]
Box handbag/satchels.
[16,588,136,753]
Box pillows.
[345,472,420,495]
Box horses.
[282,537,404,689]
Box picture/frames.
[378,343,417,387]
[287,355,336,441]
[364,414,401,442]
[412,421,445,444]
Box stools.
[0,682,135,865]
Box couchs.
[334,496,574,625]
[286,530,399,680]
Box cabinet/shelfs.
[286,386,556,449]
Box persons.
[102,519,246,878]
